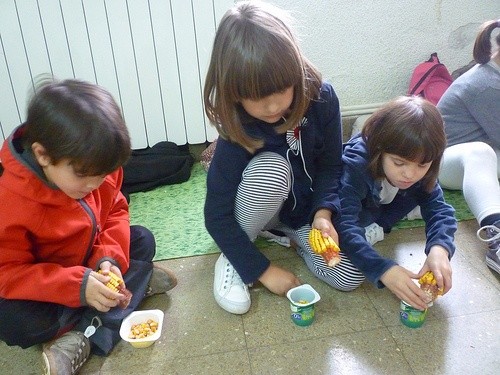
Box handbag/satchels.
[120,141,194,205]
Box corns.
[96,270,132,309]
[418,271,445,303]
[308,228,341,267]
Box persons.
[329,93,457,313]
[201,3,370,315]
[0,77,180,375]
[430,19,500,277]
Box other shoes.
[213,252,253,314]
[41,331,91,375]
[144,266,178,297]
[477,220,500,274]
[259,231,291,247]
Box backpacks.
[408,52,453,107]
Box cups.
[400,300,433,329]
[119,310,164,348]
[287,284,321,326]
